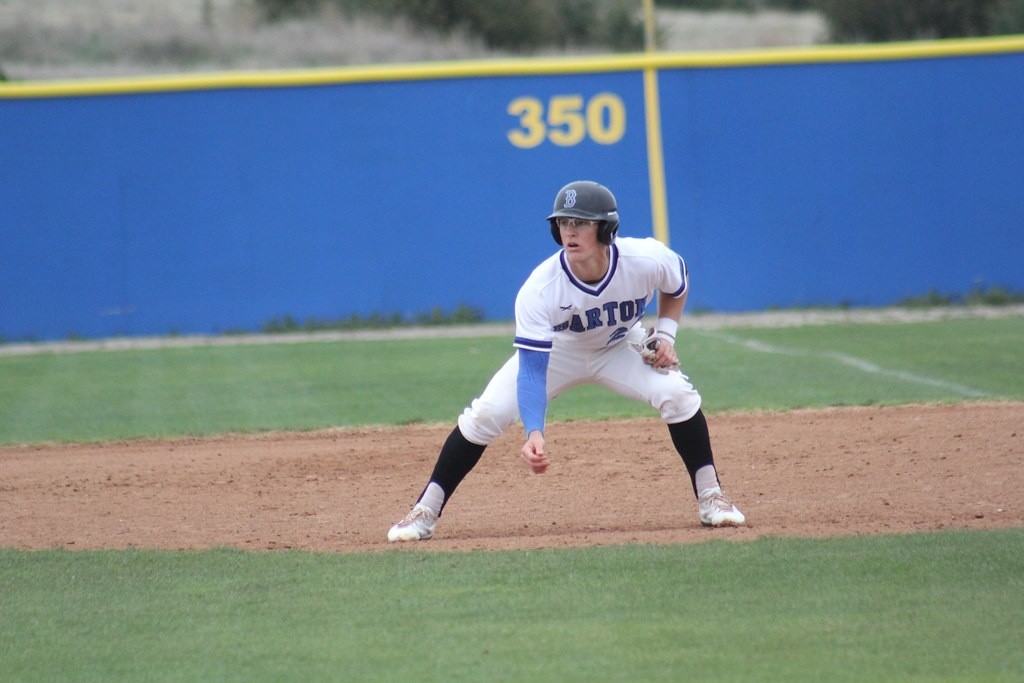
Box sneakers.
[388,505,436,543]
[698,489,745,525]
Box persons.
[387,180,746,544]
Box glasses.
[555,217,603,232]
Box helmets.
[547,181,619,247]
